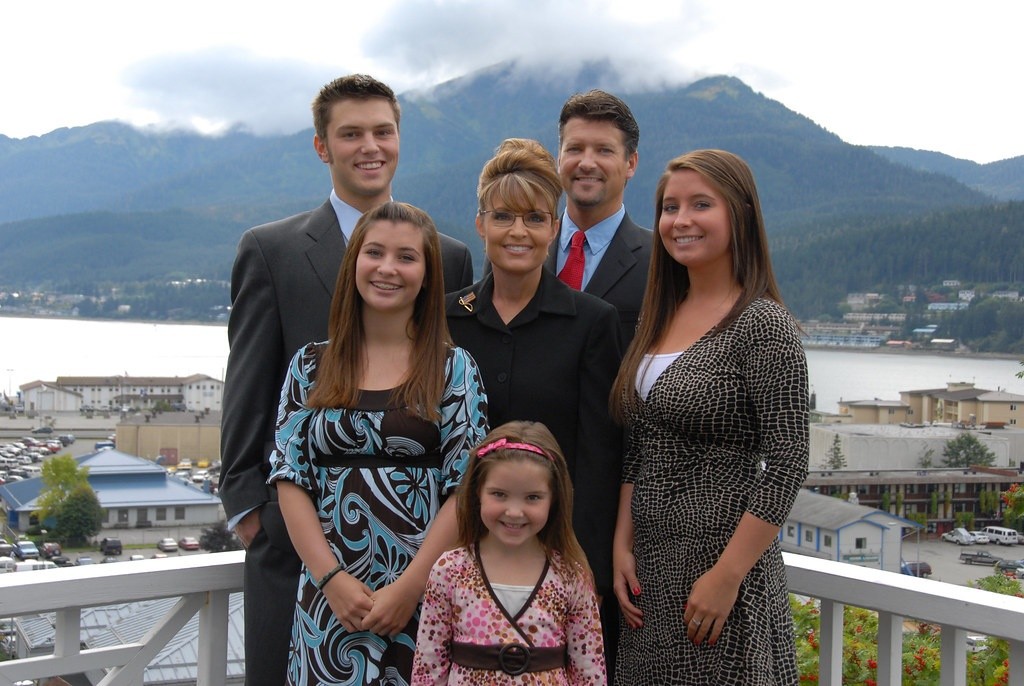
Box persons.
[545,89,655,345]
[610,150,810,686]
[444,138,618,686]
[218,74,473,686]
[410,422,607,686]
[265,200,491,686]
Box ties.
[557,231,585,291]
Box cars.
[0,434,223,573]
[99,405,112,412]
[940,525,1024,580]
[80,405,94,412]
[902,562,932,578]
[967,634,990,654]
[32,426,53,434]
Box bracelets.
[316,563,344,590]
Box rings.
[692,617,701,627]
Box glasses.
[480,208,552,229]
